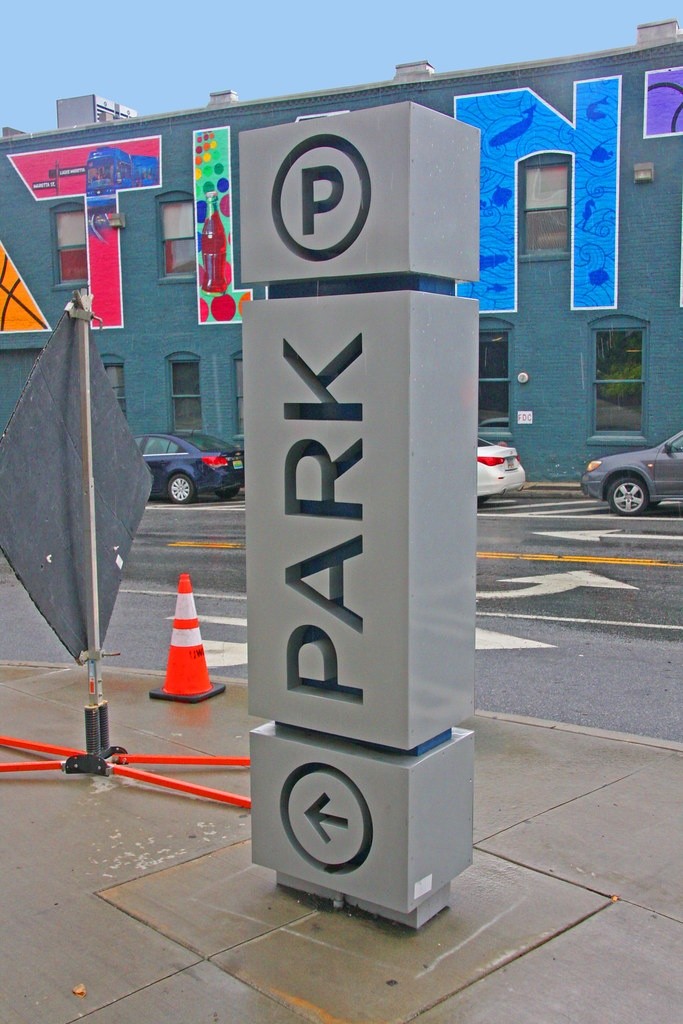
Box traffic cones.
[147,573,227,702]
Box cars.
[581,428,683,516]
[133,432,244,504]
[476,436,526,504]
